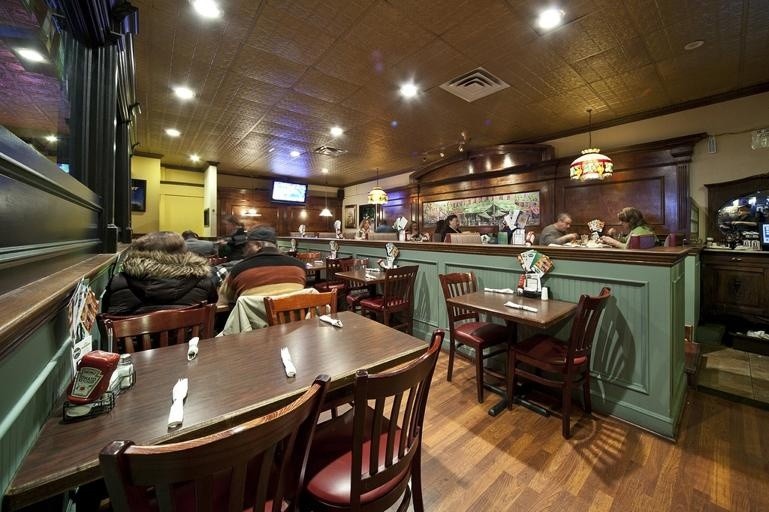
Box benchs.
[316,227,688,248]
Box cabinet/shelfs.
[701,247,769,331]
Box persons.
[443,216,461,239]
[106,231,217,315]
[601,208,657,251]
[406,221,418,238]
[538,214,578,246]
[220,228,247,258]
[184,231,216,256]
[434,220,444,235]
[218,224,306,298]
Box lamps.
[318,180,333,217]
[366,168,389,207]
[569,108,614,183]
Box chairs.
[99,372,332,512]
[294,327,446,512]
[437,270,507,403]
[506,286,612,437]
[92,250,419,358]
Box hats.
[237,224,277,246]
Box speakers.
[337,189,344,199]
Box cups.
[581,235,588,243]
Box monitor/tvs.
[759,221,769,246]
[271,180,308,206]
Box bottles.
[116,353,135,388]
[518,273,526,293]
[524,231,537,246]
[540,287,549,301]
[744,232,750,247]
[706,237,718,247]
[752,233,760,249]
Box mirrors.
[703,171,769,247]
